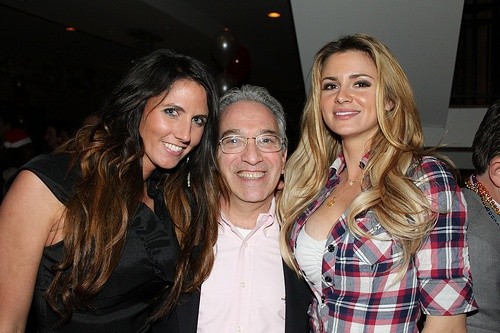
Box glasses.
[217,134,285,154]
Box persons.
[166,85,314,333]
[0,48,284,333]
[459,105,500,333]
[0,114,36,183]
[274,33,479,333]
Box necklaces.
[326,198,337,206]
[464,173,500,229]
